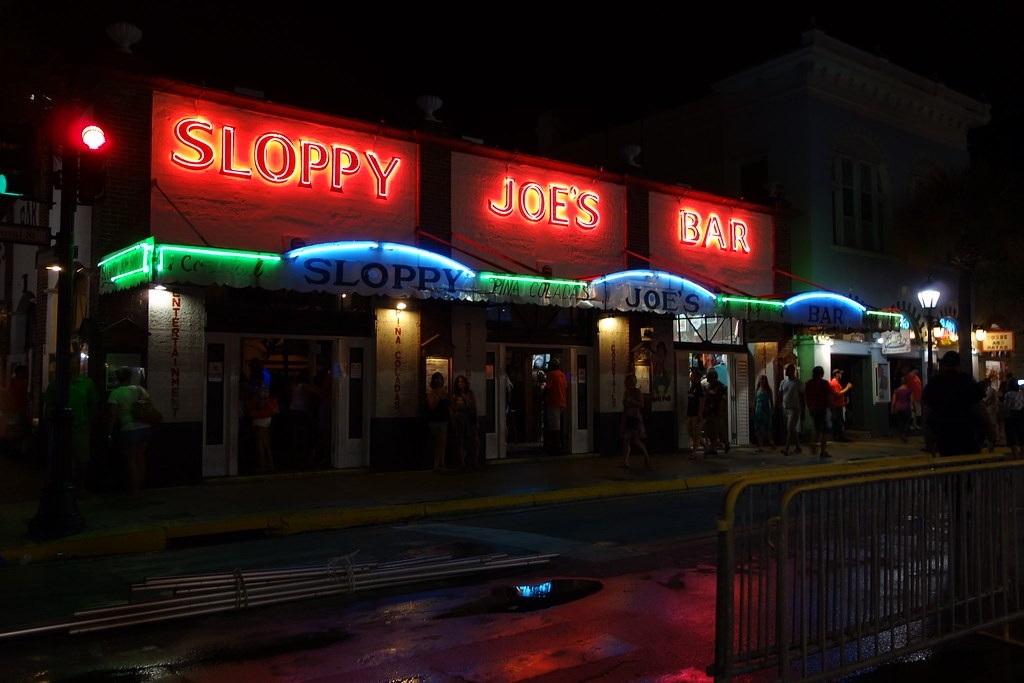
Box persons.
[46,357,98,497]
[616,373,649,468]
[8,365,32,463]
[891,367,923,442]
[779,364,852,458]
[107,369,153,499]
[687,370,731,460]
[542,358,566,451]
[245,367,333,471]
[922,351,997,526]
[505,374,514,413]
[753,375,778,452]
[428,372,484,472]
[979,373,1024,460]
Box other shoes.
[901,434,907,442]
[644,464,654,469]
[433,467,454,476]
[781,449,793,455]
[704,450,718,458]
[689,453,697,459]
[616,463,630,469]
[838,437,852,443]
[913,424,924,431]
[793,449,801,453]
[820,450,832,458]
[725,442,730,453]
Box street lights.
[918,287,941,386]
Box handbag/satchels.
[455,406,477,422]
[132,384,155,420]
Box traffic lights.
[75,120,114,156]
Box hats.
[832,369,844,375]
